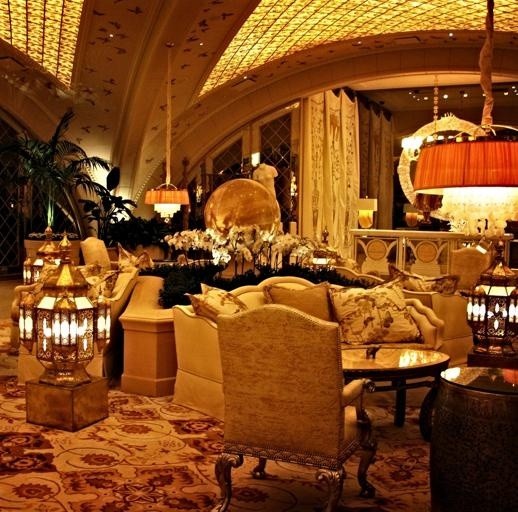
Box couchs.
[172,275,446,422]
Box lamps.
[465,239,518,369]
[145,41,190,223]
[415,1,518,198]
[18,227,115,388]
[356,198,377,228]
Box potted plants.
[12,102,138,290]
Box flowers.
[161,225,312,267]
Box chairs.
[208,305,376,512]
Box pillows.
[264,282,424,345]
[186,282,250,325]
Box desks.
[431,366,518,511]
